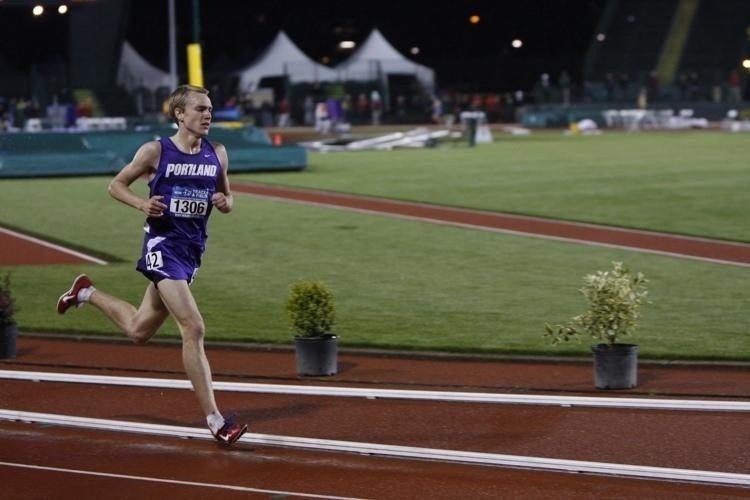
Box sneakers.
[212,420,248,448]
[56,273,94,314]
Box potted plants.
[538,258,655,391]
[0,269,23,359]
[285,276,341,377]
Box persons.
[1,81,243,128]
[56,83,249,447]
[531,64,748,106]
[246,91,534,133]
